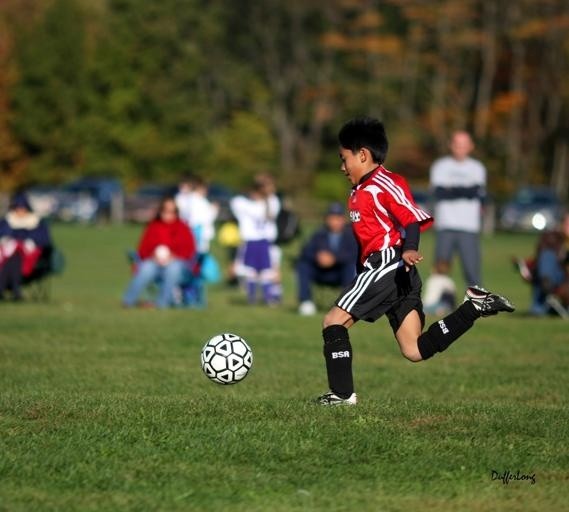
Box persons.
[122,170,353,309]
[316,114,516,407]
[510,211,569,320]
[427,129,486,289]
[0,189,51,300]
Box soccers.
[201,334,254,385]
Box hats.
[327,202,344,214]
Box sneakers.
[315,390,357,406]
[299,300,315,315]
[464,285,515,316]
